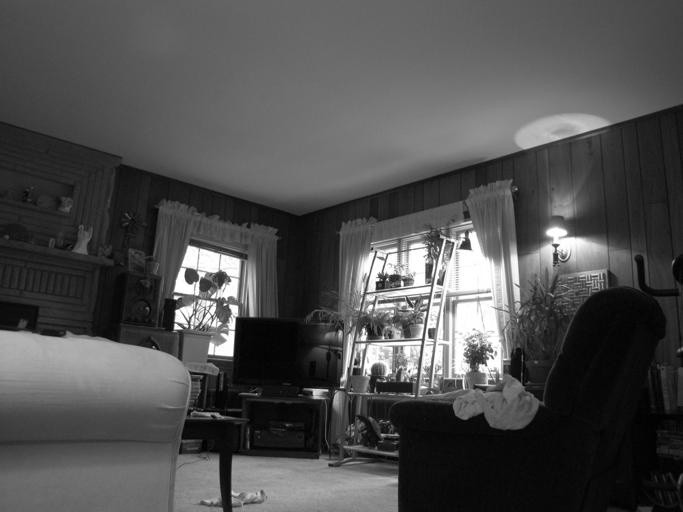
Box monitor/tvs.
[231,317,345,399]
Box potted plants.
[490,264,582,385]
[419,221,457,286]
[173,264,247,365]
[323,287,441,341]
[374,263,414,290]
[461,327,499,389]
[347,352,441,392]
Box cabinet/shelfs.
[239,394,325,460]
[326,229,457,469]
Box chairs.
[388,284,666,512]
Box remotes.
[237,392,261,398]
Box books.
[642,361,682,512]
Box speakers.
[110,271,164,327]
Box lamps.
[542,215,571,264]
